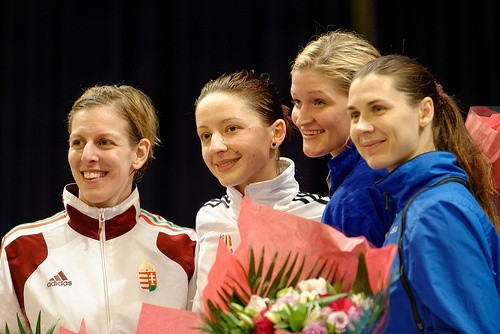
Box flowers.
[137,195,399,334]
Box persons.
[290,30,394,248]
[0,84,197,334]
[191,67,332,311]
[345,55,500,334]
